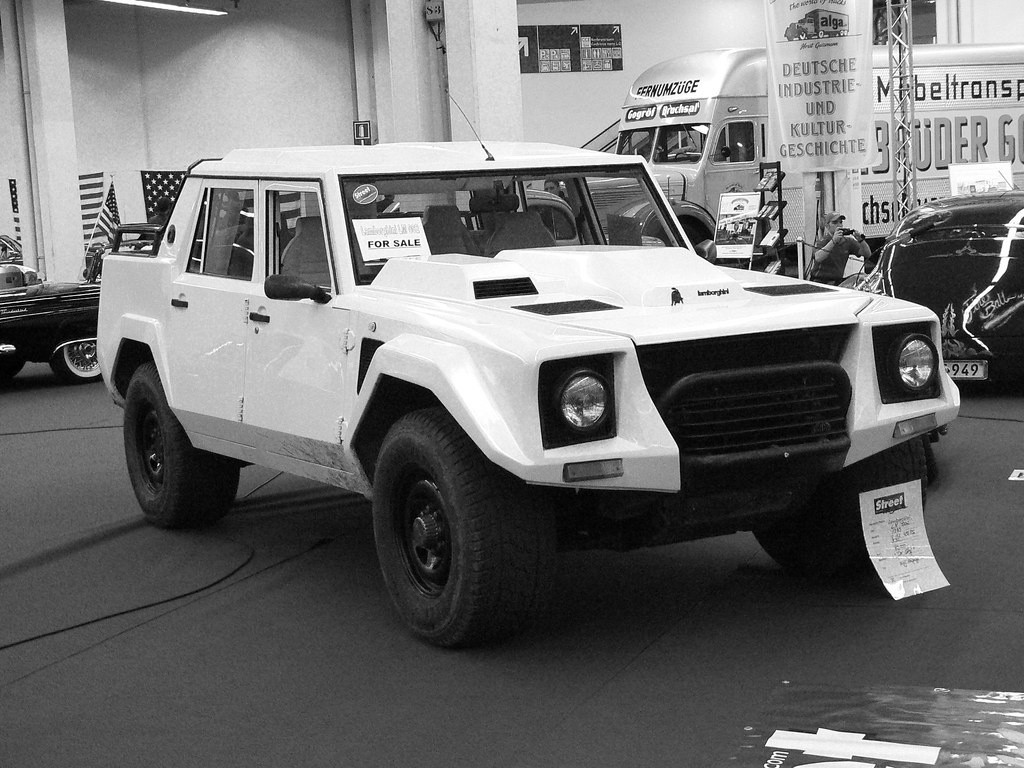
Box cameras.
[839,228,854,235]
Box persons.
[808,211,871,288]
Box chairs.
[484,211,556,256]
[279,215,371,287]
[422,204,483,256]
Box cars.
[377,189,666,247]
[881,190,1024,396]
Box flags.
[140,170,301,231]
[96,178,125,245]
[8,170,108,245]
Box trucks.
[559,44,1024,265]
[0,223,254,381]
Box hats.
[826,211,846,224]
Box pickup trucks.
[97,144,960,646]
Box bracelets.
[831,238,837,244]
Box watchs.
[858,233,866,243]
